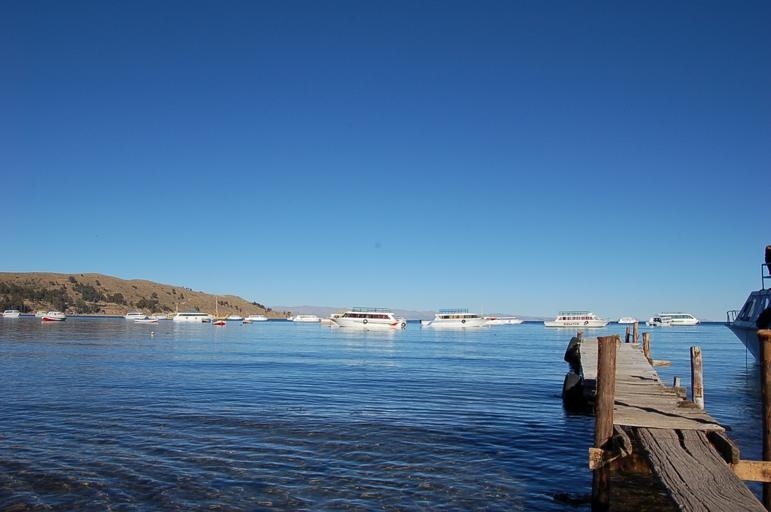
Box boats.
[286,308,407,329]
[645,313,700,328]
[123,312,226,326]
[3,310,21,318]
[618,316,639,324]
[544,311,609,329]
[35,311,67,321]
[227,314,268,324]
[420,308,524,330]
